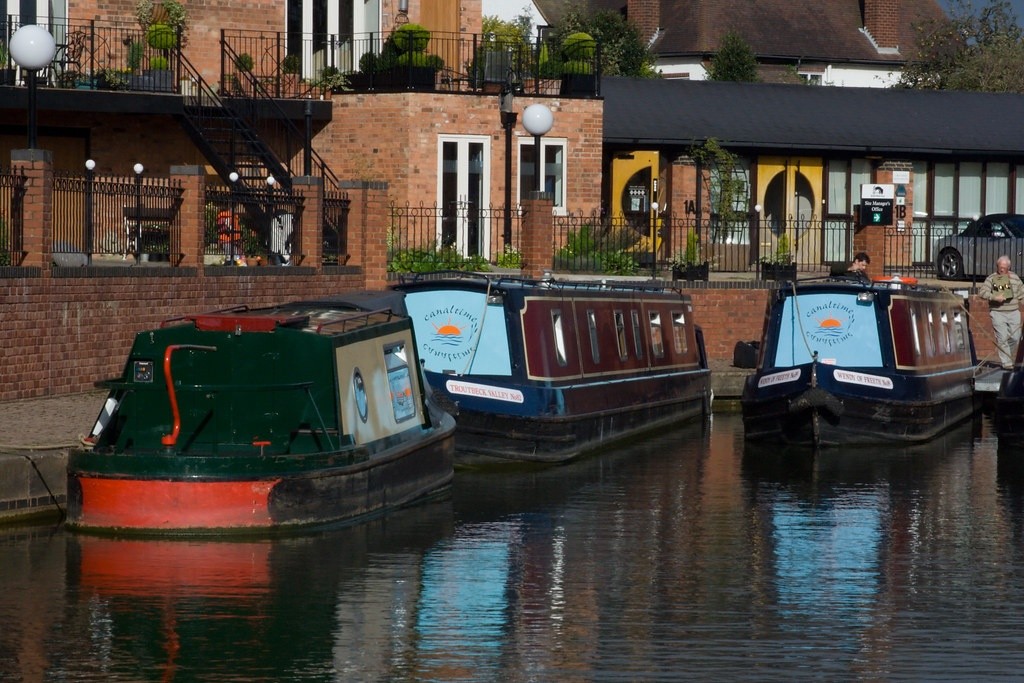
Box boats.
[972,321,1024,410]
[733,274,982,445]
[387,268,715,462]
[59,301,457,537]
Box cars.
[932,214,1024,284]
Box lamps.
[398,0,409,12]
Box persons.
[829,253,871,282]
[978,256,1024,370]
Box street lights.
[10,23,58,150]
[520,102,556,197]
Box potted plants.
[128,223,173,260]
[760,233,797,282]
[235,221,269,265]
[553,225,656,273]
[222,53,339,101]
[467,30,508,95]
[0,39,17,85]
[516,33,596,98]
[340,21,446,93]
[671,227,709,284]
[56,70,78,89]
[97,25,176,89]
[135,1,188,46]
